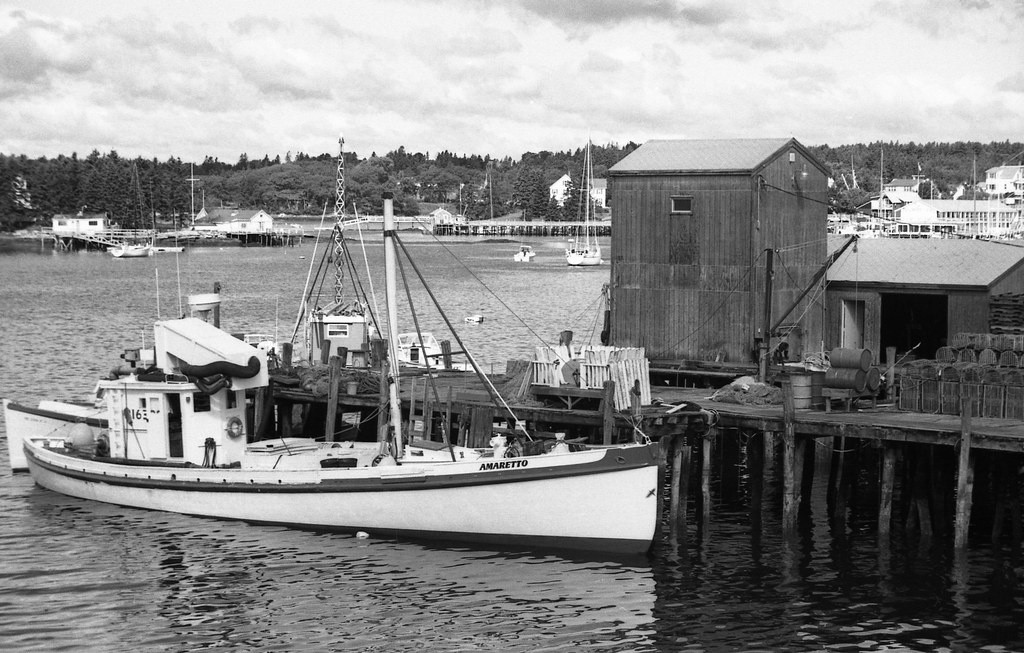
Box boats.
[0,204,668,558]
[514,244,538,263]
[150,243,186,254]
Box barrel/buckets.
[810,372,826,410]
[825,348,880,392]
[790,372,812,411]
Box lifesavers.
[227,417,243,438]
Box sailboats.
[564,137,604,267]
[105,163,153,259]
